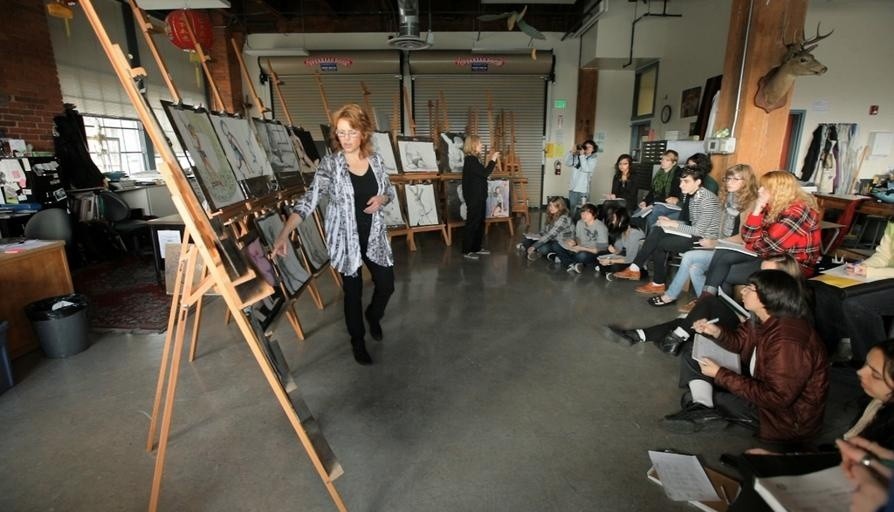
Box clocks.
[662,105,671,124]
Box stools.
[664,252,682,268]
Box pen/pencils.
[693,242,700,244]
[721,485,731,506]
[690,318,719,329]
[860,452,871,467]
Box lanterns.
[164,10,215,88]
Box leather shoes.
[679,301,696,314]
[606,325,643,347]
[352,343,373,366]
[463,251,480,260]
[366,310,383,341]
[515,243,665,296]
[648,295,676,306]
[474,248,491,257]
[831,356,865,377]
[654,331,684,357]
[658,402,729,435]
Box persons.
[491,186,506,217]
[288,129,317,171]
[461,135,500,259]
[602,254,894,512]
[222,119,254,175]
[188,123,216,179]
[516,137,822,302]
[811,218,894,366]
[266,103,396,366]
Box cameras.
[576,144,587,150]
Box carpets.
[75,264,218,334]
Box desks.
[0,184,185,363]
[814,193,894,258]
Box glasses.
[722,176,745,184]
[335,129,359,139]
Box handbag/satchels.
[866,179,894,203]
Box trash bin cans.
[23,293,92,359]
